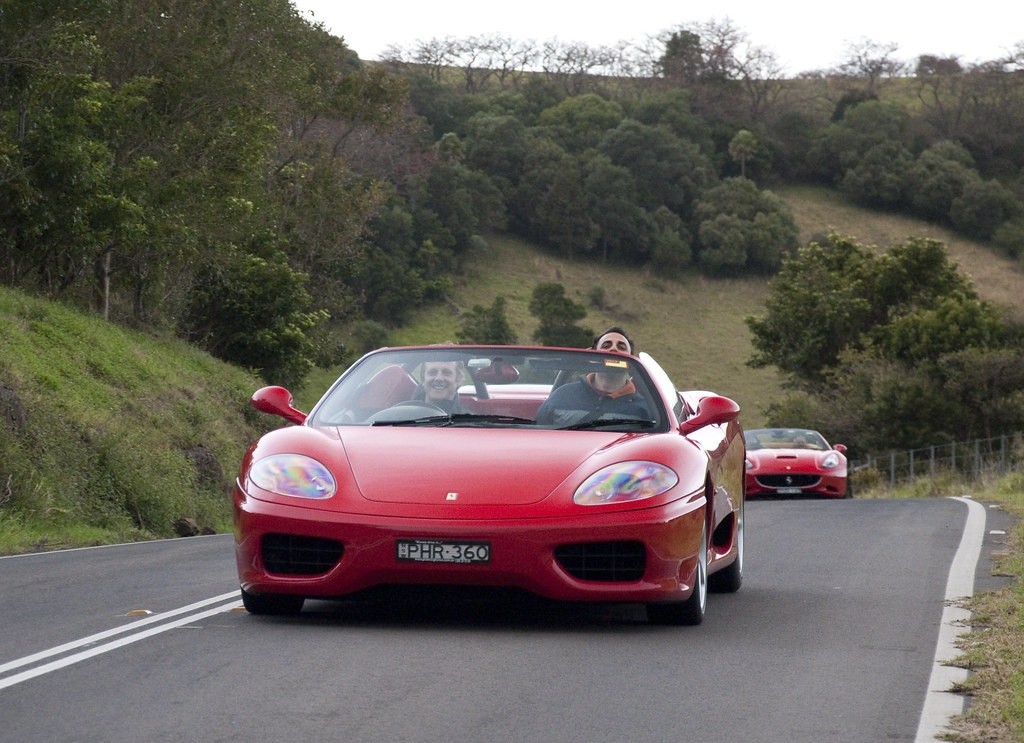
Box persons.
[793,436,809,449]
[530,327,655,432]
[410,343,472,418]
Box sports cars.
[742,428,852,502]
[230,343,747,627]
